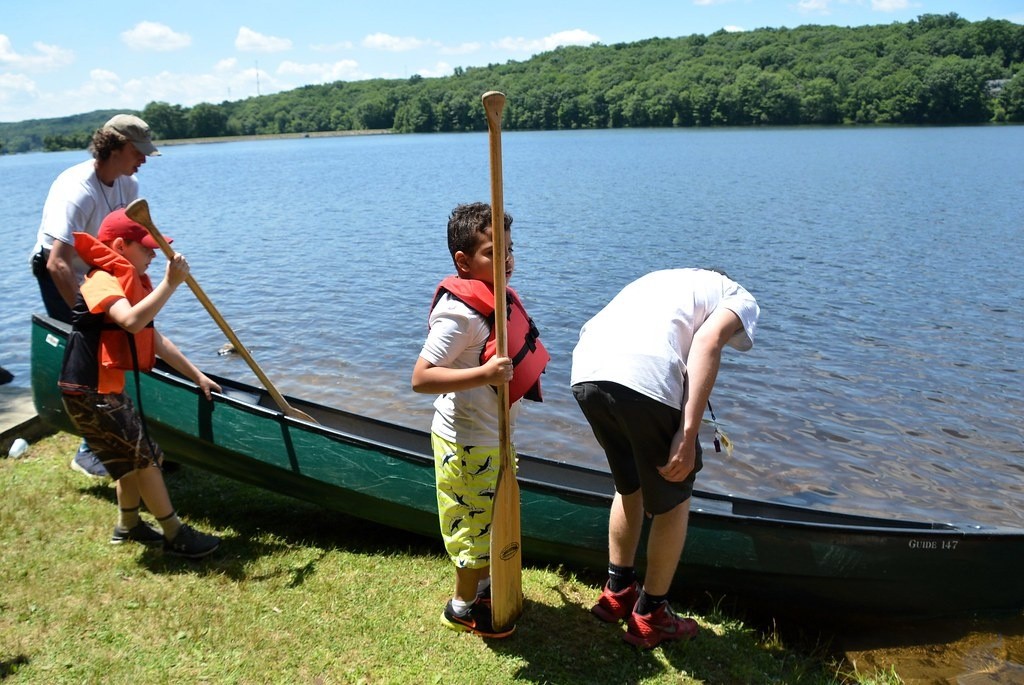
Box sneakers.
[590,579,641,624]
[109,514,166,545]
[479,584,525,607]
[439,599,518,639]
[621,596,700,649]
[162,523,222,557]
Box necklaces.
[97,176,123,212]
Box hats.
[104,112,163,156]
[95,208,174,249]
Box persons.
[28,113,220,558]
[570,267,761,647]
[412,201,526,638]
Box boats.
[30,314,1024,594]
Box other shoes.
[70,447,111,479]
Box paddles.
[482,90,526,634]
[124,197,322,428]
